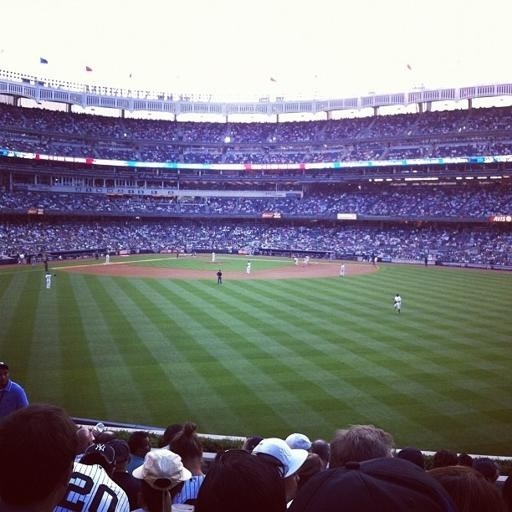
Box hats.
[285,433,312,449]
[132,450,192,482]
[252,437,309,480]
[76,423,131,463]
[290,456,450,511]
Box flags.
[86,66,93,72]
[40,57,48,64]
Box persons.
[44,272,53,289]
[1,103,511,271]
[339,263,345,278]
[245,260,251,274]
[392,292,401,315]
[216,270,223,285]
[0,360,509,511]
